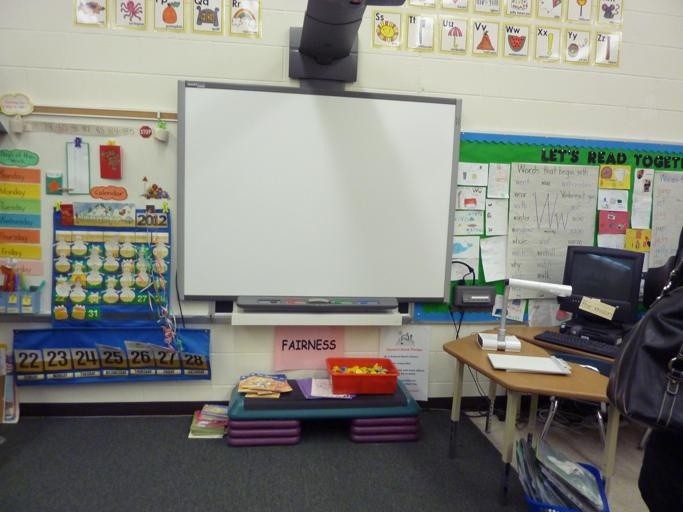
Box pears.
[162,3,177,23]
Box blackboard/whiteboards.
[179,80,461,307]
[0,102,683,328]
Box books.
[295,376,357,399]
[486,353,570,375]
[234,369,293,401]
[184,401,231,441]
[504,369,545,374]
[513,433,604,511]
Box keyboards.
[534,331,620,358]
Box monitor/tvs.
[557,245,645,329]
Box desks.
[443,329,619,505]
[508,324,619,431]
[228,378,423,446]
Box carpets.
[0,407,548,512]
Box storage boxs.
[327,357,400,395]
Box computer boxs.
[559,322,634,346]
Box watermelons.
[508,35,526,52]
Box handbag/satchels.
[607,285,683,432]
